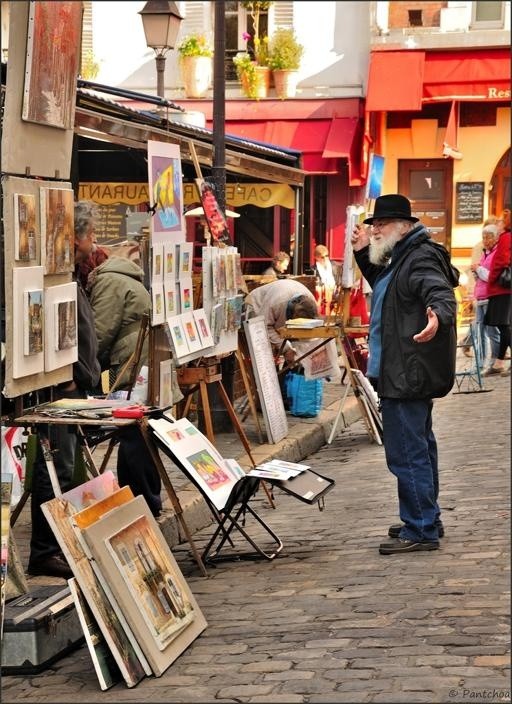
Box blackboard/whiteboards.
[455,181,485,224]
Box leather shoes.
[379,539,439,553]
[389,524,444,538]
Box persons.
[308,244,345,291]
[261,250,291,276]
[243,278,320,413]
[349,193,459,556]
[463,204,512,377]
[26,199,104,581]
[73,242,164,520]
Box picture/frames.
[81,495,209,678]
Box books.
[284,317,325,330]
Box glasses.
[372,220,390,228]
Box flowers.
[231,32,270,103]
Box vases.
[240,67,271,98]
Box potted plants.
[176,33,214,99]
[272,27,305,98]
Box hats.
[363,195,419,224]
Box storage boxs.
[0,581,85,679]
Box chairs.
[142,409,338,565]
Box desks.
[2,398,176,529]
[241,325,371,448]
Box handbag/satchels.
[499,266,512,288]
[285,372,321,417]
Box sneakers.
[460,340,473,357]
[500,367,512,377]
[28,555,73,578]
[480,364,504,377]
